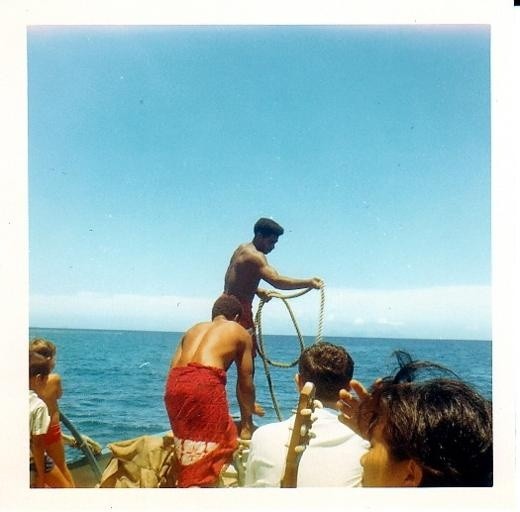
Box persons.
[337,347,492,487]
[222,217,324,440]
[30,337,77,488]
[163,293,267,489]
[29,351,71,487]
[243,341,372,487]
